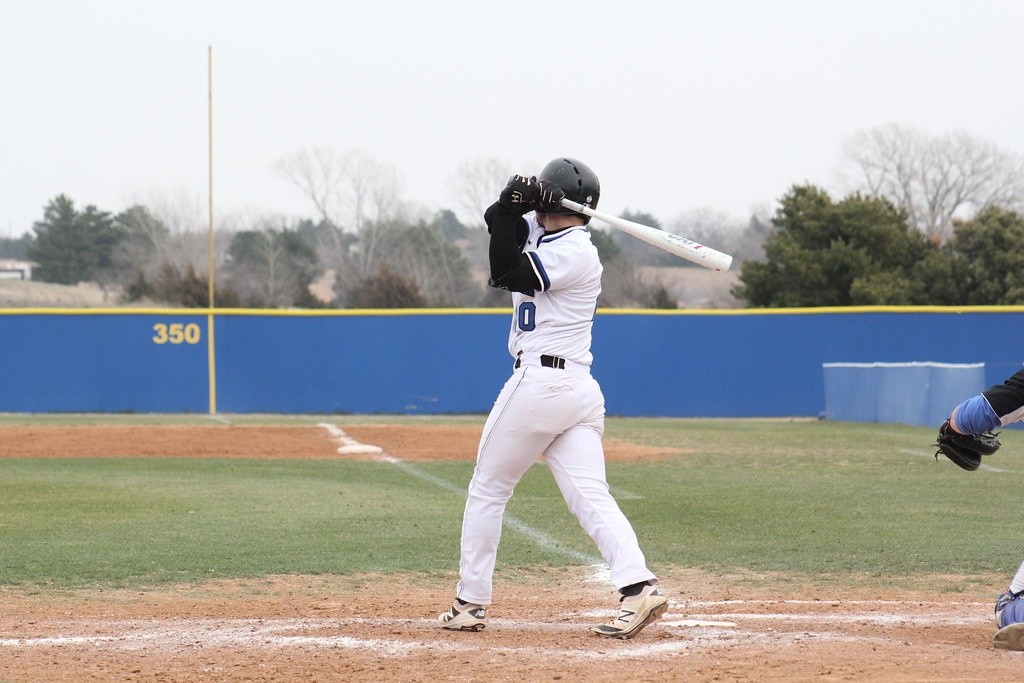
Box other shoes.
[992,622,1024,650]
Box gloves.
[537,179,565,205]
[498,174,540,216]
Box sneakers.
[437,600,487,632]
[593,585,669,639]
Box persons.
[936,368,1024,650]
[436,157,668,638]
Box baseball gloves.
[931,418,1004,471]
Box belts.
[515,354,565,369]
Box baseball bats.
[560,196,733,276]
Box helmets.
[535,157,600,224]
